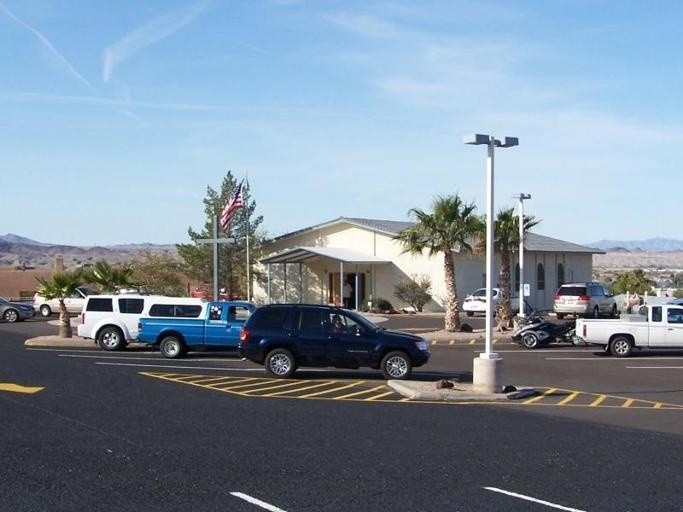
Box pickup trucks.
[136,300,256,358]
[574,303,683,358]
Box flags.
[218,180,244,234]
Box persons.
[342,279,352,306]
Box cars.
[32,286,99,317]
[553,282,618,319]
[237,302,429,379]
[462,287,527,316]
[0,295,35,324]
[75,293,220,351]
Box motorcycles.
[512,320,586,349]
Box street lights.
[463,132,520,359]
[511,192,531,318]
